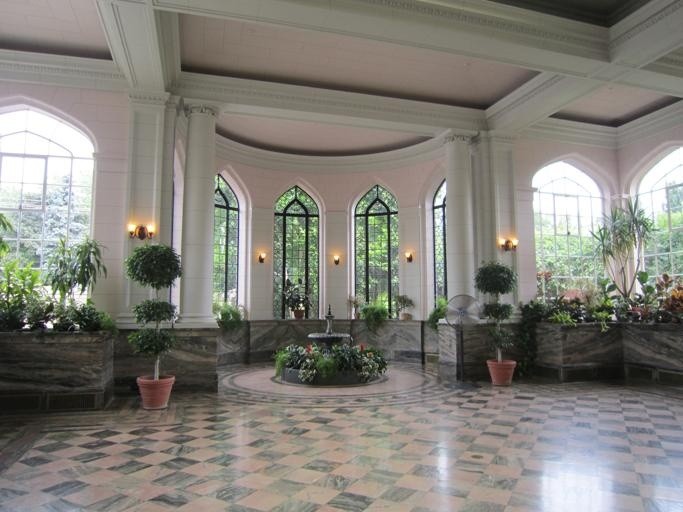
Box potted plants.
[0,214,120,410]
[473,260,520,386]
[518,196,683,383]
[281,277,313,319]
[352,295,414,331]
[123,243,183,410]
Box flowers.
[274,343,387,384]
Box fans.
[444,294,481,383]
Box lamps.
[334,256,339,265]
[259,253,267,263]
[406,252,412,263]
[128,222,155,240]
[499,238,518,251]
